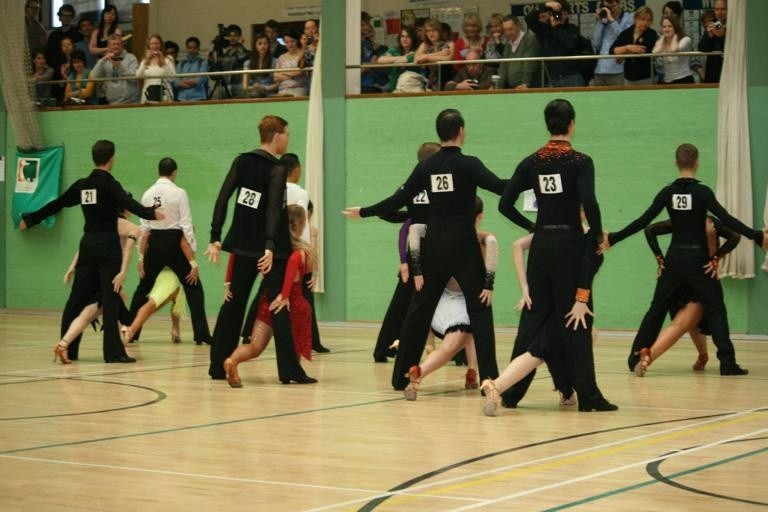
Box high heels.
[693,353,709,371]
[404,366,421,401]
[634,348,652,376]
[465,368,479,390]
[223,358,243,388]
[480,377,501,416]
[54,339,72,364]
[171,327,182,343]
[120,325,133,347]
[389,340,399,351]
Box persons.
[651,16,697,85]
[30,54,56,106]
[439,22,450,41]
[18,141,166,363]
[256,20,289,59]
[443,51,499,90]
[376,26,428,94]
[271,29,310,96]
[373,142,472,367]
[660,1,684,35]
[172,37,212,102]
[511,202,602,331]
[223,205,313,388]
[50,4,80,60]
[52,189,142,364]
[591,0,638,85]
[72,18,103,67]
[306,199,318,291]
[360,9,374,94]
[413,19,455,91]
[595,142,768,376]
[128,157,216,347]
[64,61,97,106]
[700,12,717,35]
[82,34,143,105]
[525,1,587,88]
[205,115,319,386]
[53,35,81,89]
[402,195,498,402]
[498,100,619,412]
[120,221,197,348]
[413,19,426,45]
[161,40,178,65]
[208,23,252,99]
[497,14,545,91]
[365,26,388,56]
[237,153,330,355]
[483,12,510,60]
[242,33,281,98]
[632,199,741,376]
[88,5,126,56]
[609,6,660,85]
[301,20,315,40]
[135,33,178,104]
[341,109,538,396]
[298,27,319,94]
[699,2,727,83]
[25,0,49,52]
[455,13,489,59]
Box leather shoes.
[105,350,136,363]
[313,343,330,353]
[209,360,226,379]
[282,365,317,384]
[579,399,618,411]
[720,361,748,375]
[197,335,213,344]
[501,399,517,407]
[375,357,388,362]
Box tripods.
[206,50,232,99]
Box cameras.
[599,8,605,17]
[712,19,724,30]
[111,53,120,59]
[552,11,559,21]
[213,24,229,49]
